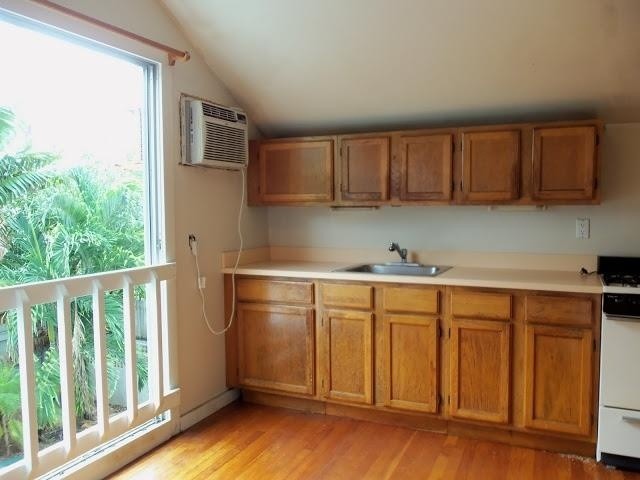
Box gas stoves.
[597,256,640,319]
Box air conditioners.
[182,96,249,171]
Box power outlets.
[576,219,590,238]
[186,232,200,249]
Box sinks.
[338,260,453,277]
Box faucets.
[388,241,407,263]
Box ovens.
[596,319,640,470]
